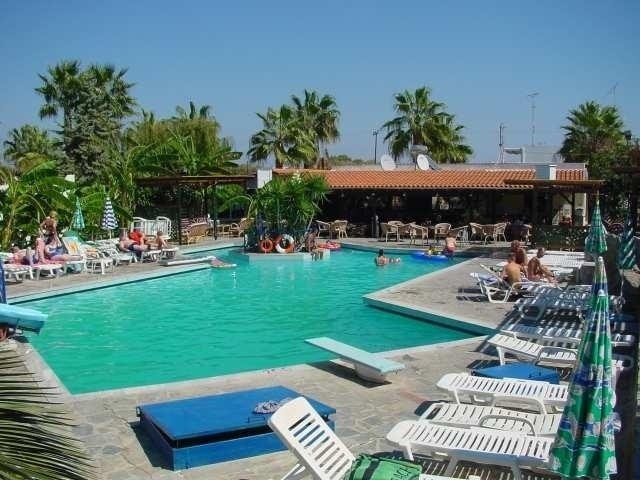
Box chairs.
[267,396,467,480]
[386,324,636,480]
[380,220,508,246]
[316,218,349,241]
[469,248,625,321]
[179,222,209,246]
[0,236,179,284]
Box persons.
[44,238,82,260]
[501,252,527,289]
[38,216,58,240]
[568,216,572,226]
[70,240,97,257]
[128,231,148,245]
[11,245,47,266]
[145,231,168,249]
[528,247,555,282]
[509,240,528,266]
[559,216,569,225]
[305,226,323,261]
[119,232,150,251]
[374,248,387,266]
[445,232,456,260]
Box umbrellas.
[547,287,621,480]
[68,198,85,235]
[585,258,609,330]
[616,207,637,269]
[585,195,608,255]
[101,195,119,239]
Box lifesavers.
[318,243,341,251]
[411,251,448,262]
[260,239,273,253]
[274,233,295,254]
